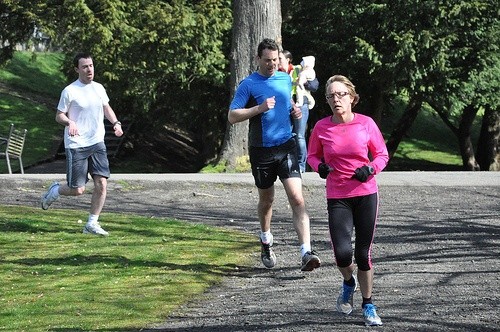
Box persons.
[227,38,320,272]
[294,55,316,110]
[41,53,124,236]
[307,75,389,325]
[276,50,308,172]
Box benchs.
[0,124,27,174]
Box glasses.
[326,91,351,101]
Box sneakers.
[41,183,62,210]
[260,232,277,268]
[82,221,110,238]
[361,302,383,327]
[336,275,358,315]
[301,249,322,272]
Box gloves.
[318,163,333,180]
[351,166,372,182]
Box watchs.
[367,166,375,175]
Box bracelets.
[113,121,122,126]
